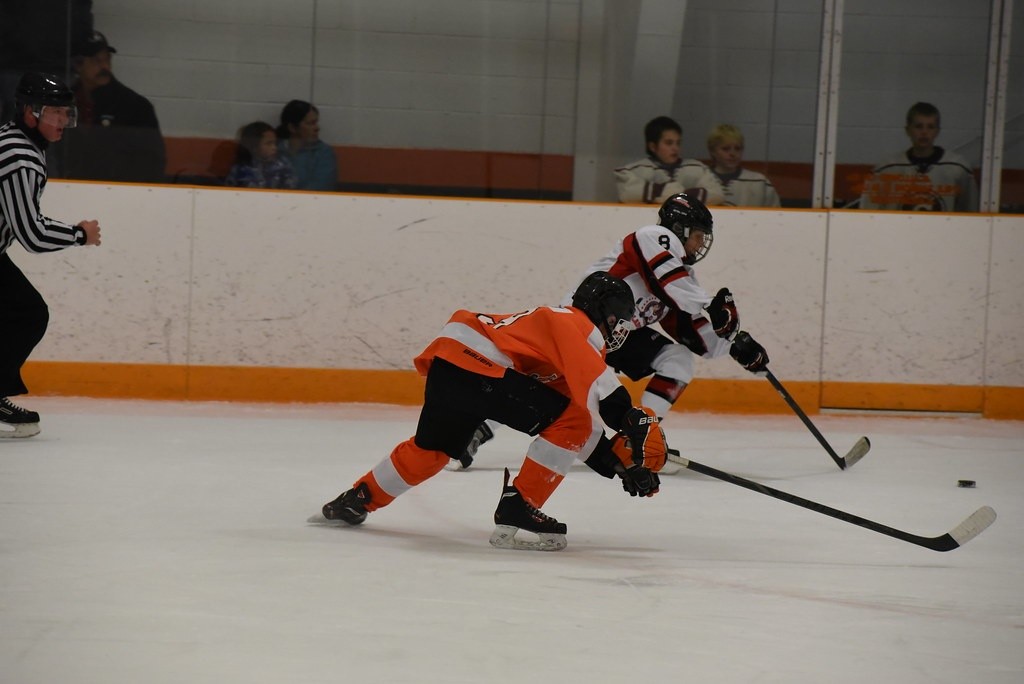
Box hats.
[77,29,116,58]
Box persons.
[613,116,724,205]
[307,271,668,550]
[861,102,980,213]
[707,123,780,207]
[225,100,336,191]
[444,192,769,475]
[0,70,103,438]
[44,30,167,181]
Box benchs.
[162,135,576,202]
[698,160,1024,217]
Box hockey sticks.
[730,331,873,471]
[662,453,999,553]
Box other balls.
[957,479,976,489]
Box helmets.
[13,70,72,115]
[569,271,635,352]
[659,189,714,265]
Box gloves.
[708,284,737,339]
[621,407,670,472]
[619,465,660,497]
[729,330,770,376]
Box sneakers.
[661,449,681,474]
[0,397,40,438]
[489,467,569,550]
[437,420,493,472]
[308,481,372,527]
